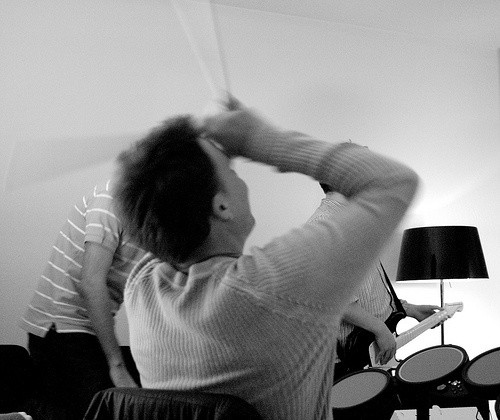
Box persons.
[301,145,450,372]
[109,92,421,420]
[17,170,146,420]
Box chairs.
[83,388,261,420]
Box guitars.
[344,302,463,372]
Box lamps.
[395,226,489,345]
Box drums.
[462,347,500,400]
[395,344,469,408]
[333,369,396,420]
